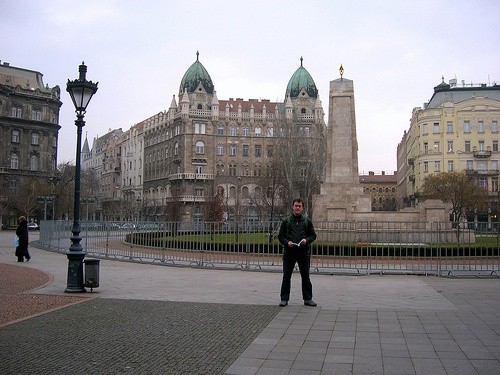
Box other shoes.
[304,300,317,307]
[279,301,288,307]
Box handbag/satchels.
[13,235,19,246]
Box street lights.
[65,60,99,294]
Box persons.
[15,216,31,263]
[277,198,317,307]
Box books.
[291,239,304,247]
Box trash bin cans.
[83,258,100,288]
